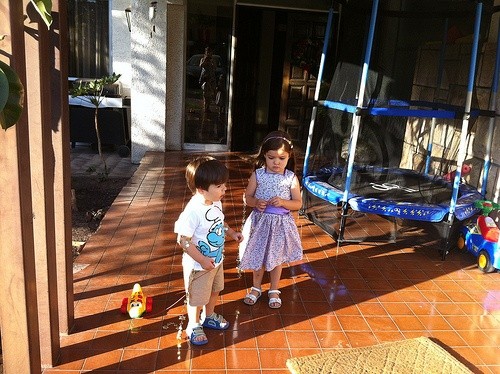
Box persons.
[238,130,304,309]
[199,49,217,114]
[173,155,243,346]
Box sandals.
[200,311,229,330]
[186,323,208,346]
[267,290,281,309]
[243,287,262,306]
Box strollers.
[457,200,500,273]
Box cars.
[186,55,225,86]
